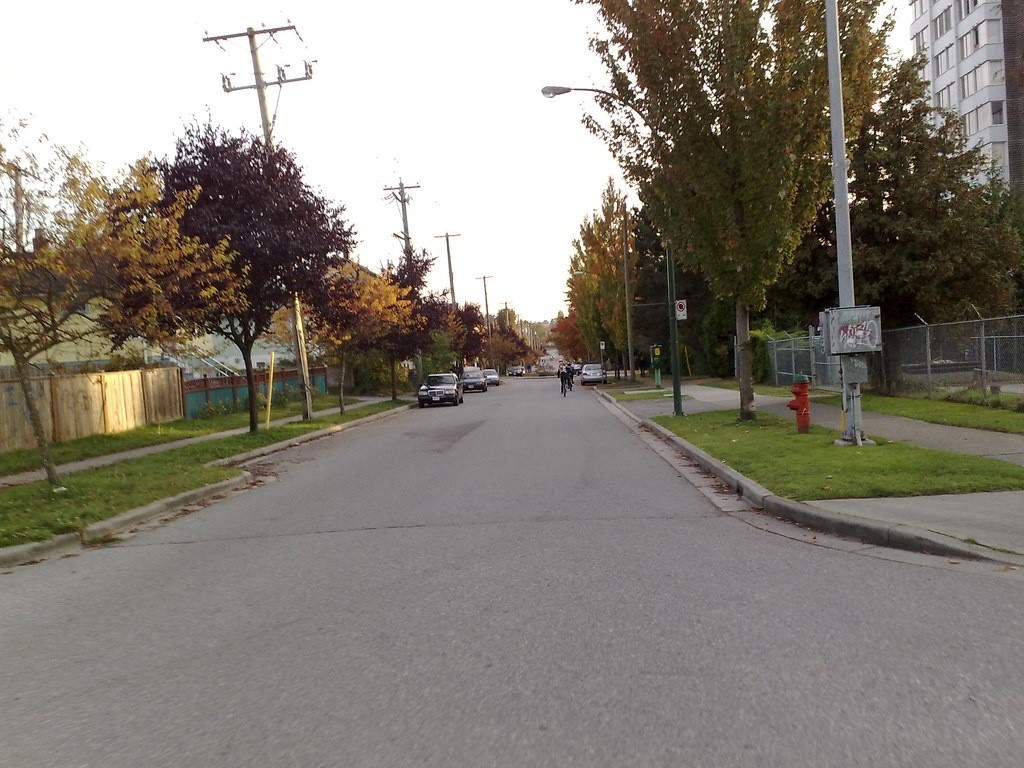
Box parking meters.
[650,344,662,388]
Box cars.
[579,363,607,385]
[481,369,500,387]
[460,370,488,392]
[507,366,524,376]
[417,373,465,409]
[571,364,582,375]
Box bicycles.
[562,378,570,397]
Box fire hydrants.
[786,369,810,434]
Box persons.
[639,356,648,378]
[557,359,574,393]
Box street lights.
[541,85,684,417]
